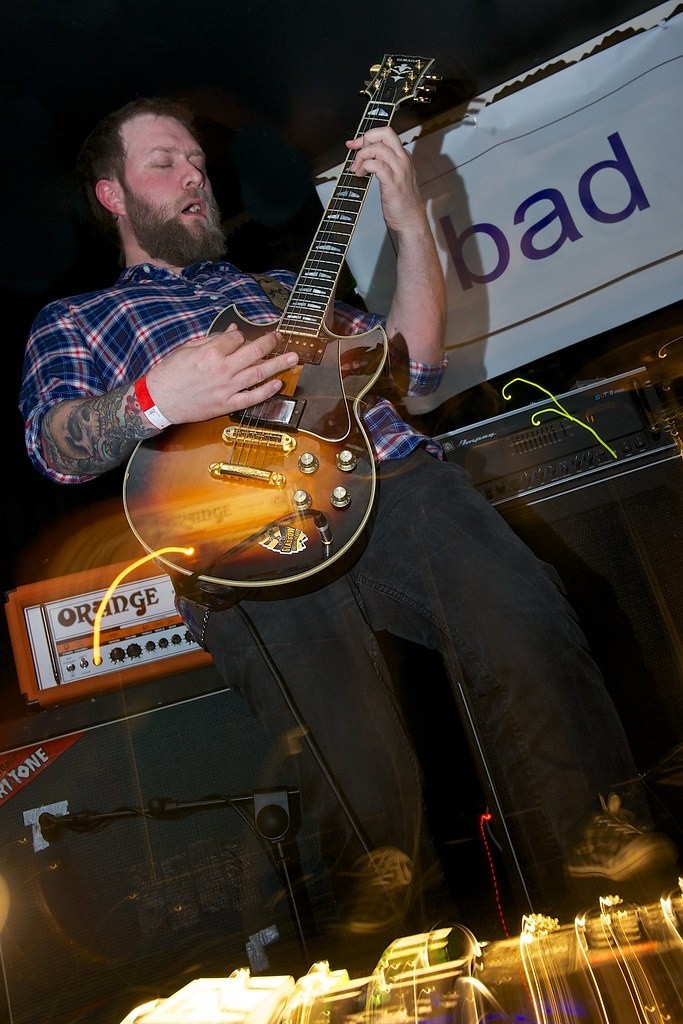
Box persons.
[19,99,676,933]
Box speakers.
[411,449,682,936]
[0,660,343,1024]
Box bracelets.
[134,375,173,431]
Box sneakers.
[561,793,677,881]
[338,844,445,933]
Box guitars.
[121,54,456,601]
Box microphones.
[39,808,90,837]
